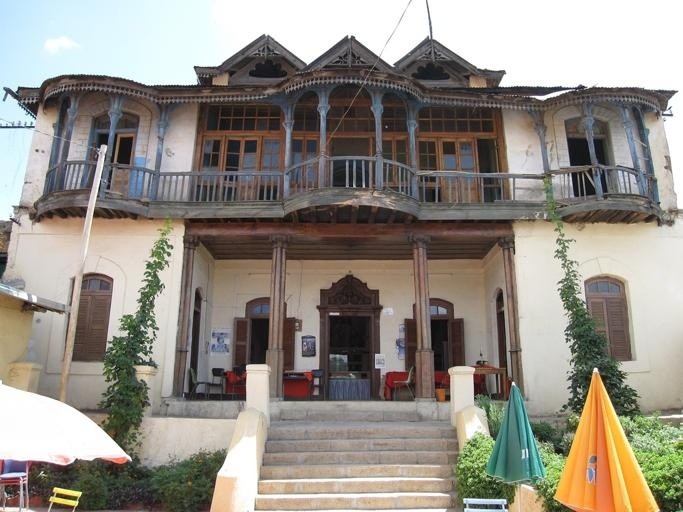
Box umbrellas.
[552,367,662,512]
[486,381,548,512]
[0,379,133,466]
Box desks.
[475,367,507,400]
[383,369,486,402]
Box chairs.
[208,366,325,402]
[391,365,416,402]
[461,498,508,512]
[46,487,81,512]
[0,460,32,512]
[188,367,211,401]
